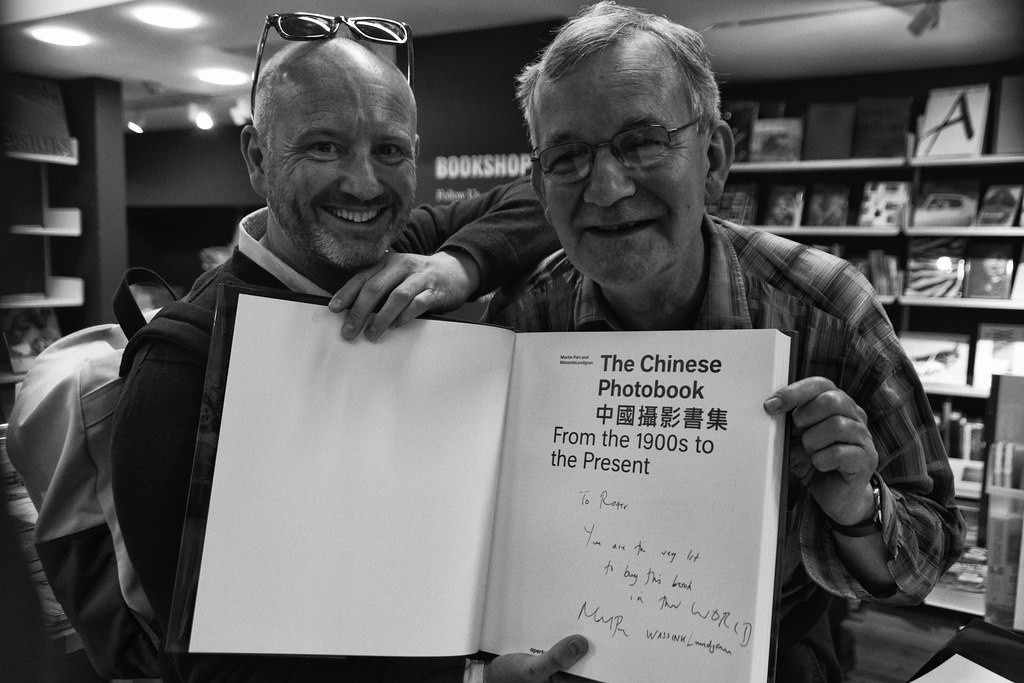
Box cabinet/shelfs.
[0,145,85,385]
[713,153,1024,503]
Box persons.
[477,1,969,683]
[107,34,563,683]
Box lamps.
[196,110,213,130]
[127,120,144,133]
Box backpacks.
[10,268,212,683]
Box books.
[0,308,62,373]
[898,324,1024,387]
[716,179,1024,227]
[163,281,800,683]
[932,400,983,500]
[811,237,1024,300]
[984,442,1022,630]
[719,74,1024,162]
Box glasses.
[530,118,704,186]
[250,11,416,123]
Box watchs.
[820,475,886,539]
